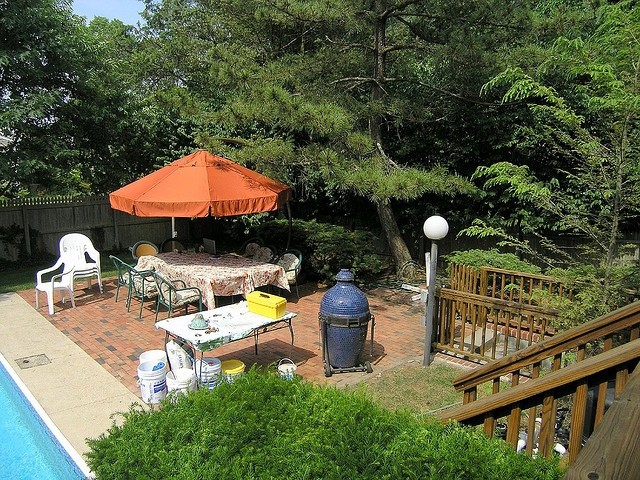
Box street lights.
[420,213,451,367]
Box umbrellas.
[109,143,289,252]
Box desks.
[155,301,297,387]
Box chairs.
[131,240,159,262]
[161,238,187,259]
[235,238,264,261]
[36,232,103,317]
[121,261,163,320]
[148,270,202,318]
[271,248,302,301]
[108,255,157,310]
[249,243,277,264]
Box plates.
[188,325,209,330]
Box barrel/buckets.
[278,358,297,380]
[166,369,197,402]
[138,350,171,372]
[137,361,166,404]
[193,357,222,390]
[222,360,246,383]
